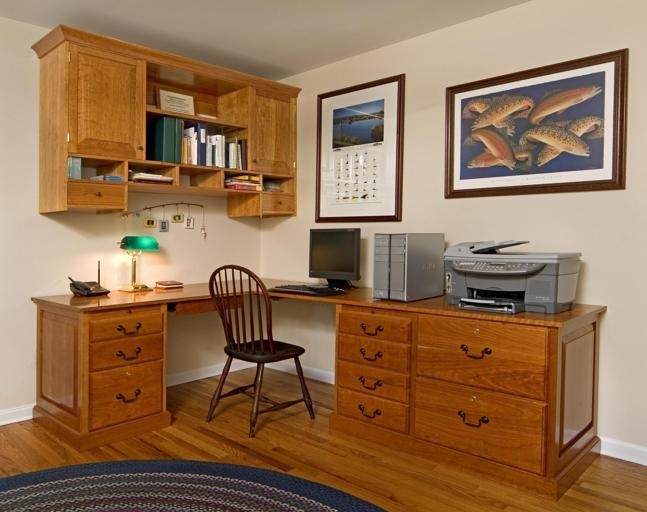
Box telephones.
[67,261,111,295]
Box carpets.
[0,456,388,511]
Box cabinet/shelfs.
[26,293,175,455]
[330,284,412,454]
[128,46,258,192]
[30,24,143,214]
[218,75,301,216]
[413,283,607,499]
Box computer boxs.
[371,232,445,302]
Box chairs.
[206,265,320,435]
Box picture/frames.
[442,47,630,199]
[314,72,404,221]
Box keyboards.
[267,285,344,296]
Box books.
[149,116,248,170]
[134,179,173,184]
[131,171,173,182]
[155,279,185,289]
[68,157,124,182]
[227,176,262,191]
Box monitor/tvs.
[309,228,361,288]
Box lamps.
[117,229,159,291]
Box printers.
[440,239,583,315]
